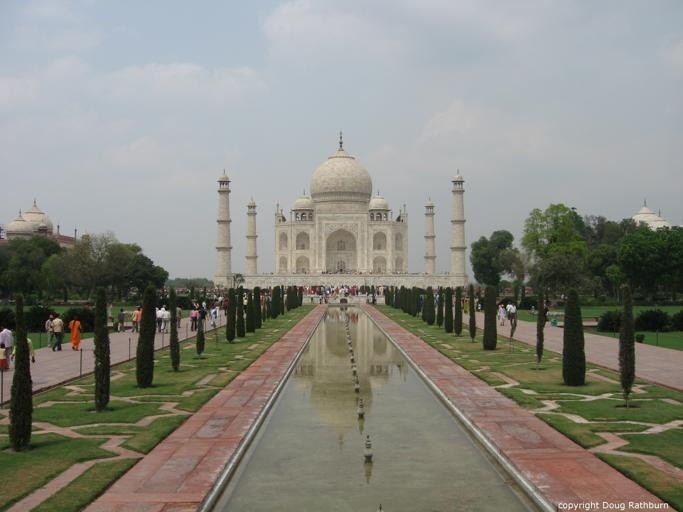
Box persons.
[0,284,549,373]
